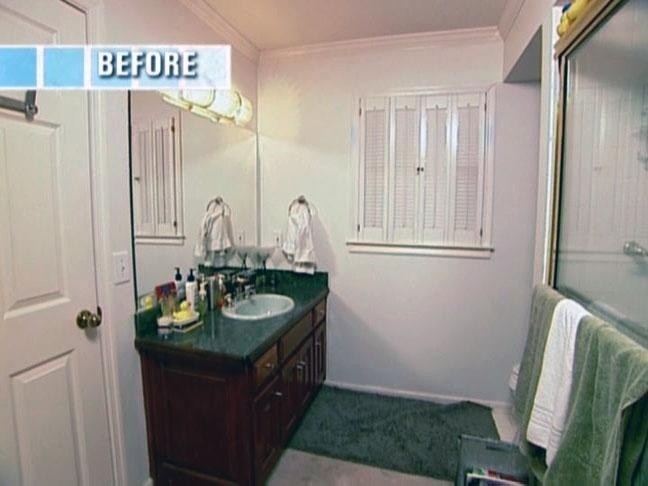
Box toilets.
[490,365,521,442]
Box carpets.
[288,377,503,486]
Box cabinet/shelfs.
[282,308,315,452]
[315,297,327,398]
[254,344,282,486]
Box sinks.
[218,292,297,321]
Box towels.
[508,281,648,486]
[279,210,319,276]
[192,211,233,260]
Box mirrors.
[126,84,262,314]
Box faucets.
[234,275,250,301]
